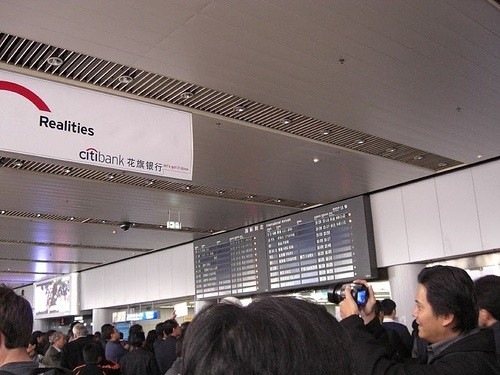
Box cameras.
[328,283,368,305]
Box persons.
[180,293,369,375]
[474,274,500,375]
[338,264,497,375]
[374,299,431,362]
[26,310,189,375]
[0,282,73,375]
